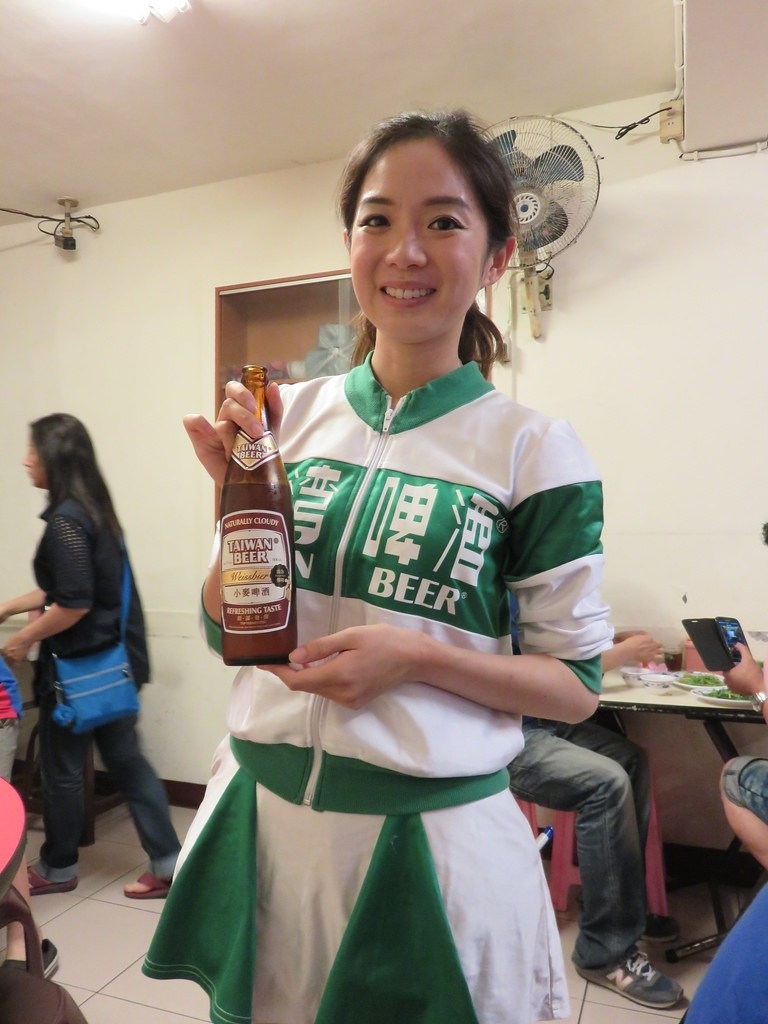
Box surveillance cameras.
[55,236,76,250]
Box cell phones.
[715,617,751,663]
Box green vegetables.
[677,672,755,701]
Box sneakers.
[638,914,679,943]
[575,950,684,1008]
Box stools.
[513,777,667,932]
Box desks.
[599,667,768,964]
[0,777,46,976]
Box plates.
[671,672,752,707]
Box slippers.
[124,871,169,899]
[26,865,78,896]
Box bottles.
[218,363,298,666]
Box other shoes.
[42,939,58,977]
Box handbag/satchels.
[53,643,140,735]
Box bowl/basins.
[621,666,672,692]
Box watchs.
[751,692,768,712]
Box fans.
[475,114,599,342]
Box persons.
[679,517,768,1024]
[0,413,183,1024]
[141,112,615,1024]
[508,586,685,1011]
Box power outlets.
[658,99,685,147]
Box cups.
[665,650,682,672]
